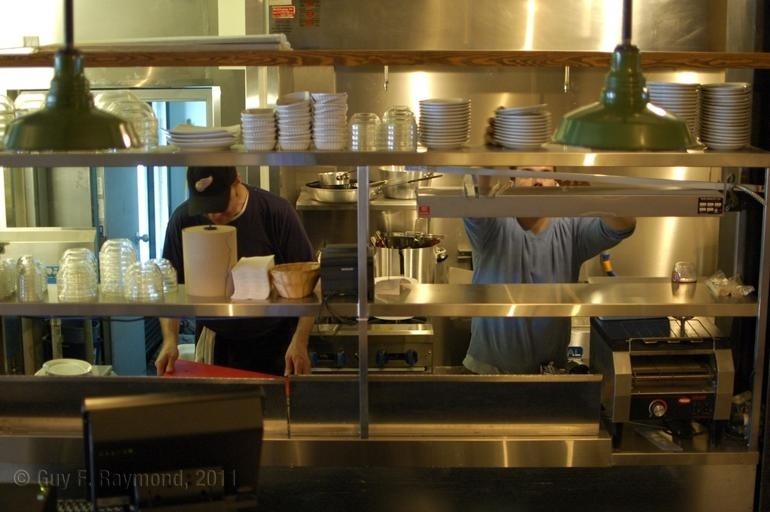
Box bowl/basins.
[351,101,419,152]
[0,238,179,303]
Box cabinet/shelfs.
[4,49,770,483]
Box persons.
[460,105,637,374]
[155,166,322,378]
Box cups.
[673,261,696,284]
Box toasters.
[587,315,736,450]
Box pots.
[307,167,444,205]
[370,230,448,288]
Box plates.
[650,80,753,149]
[42,358,94,378]
[164,114,242,152]
[420,95,472,150]
[242,87,349,153]
[493,104,552,152]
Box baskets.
[270,261,322,299]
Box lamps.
[0,0,145,150]
[546,0,710,152]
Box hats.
[186,167,236,213]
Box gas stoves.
[263,314,434,375]
